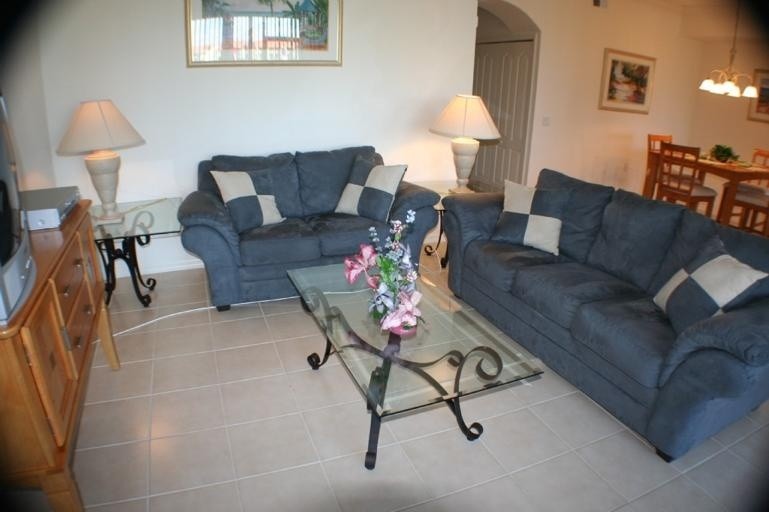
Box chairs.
[641,132,769,239]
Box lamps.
[54,97,147,222]
[697,0,761,98]
[426,93,503,196]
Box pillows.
[209,167,287,234]
[488,178,576,263]
[334,152,409,224]
[650,234,768,337]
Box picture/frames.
[746,68,769,124]
[184,0,345,68]
[595,46,657,116]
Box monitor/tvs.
[0,94,37,327]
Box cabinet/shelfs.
[0,200,121,512]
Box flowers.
[338,207,429,340]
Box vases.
[389,322,417,335]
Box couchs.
[174,144,441,312]
[440,167,769,464]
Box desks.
[82,195,186,310]
[425,193,450,268]
